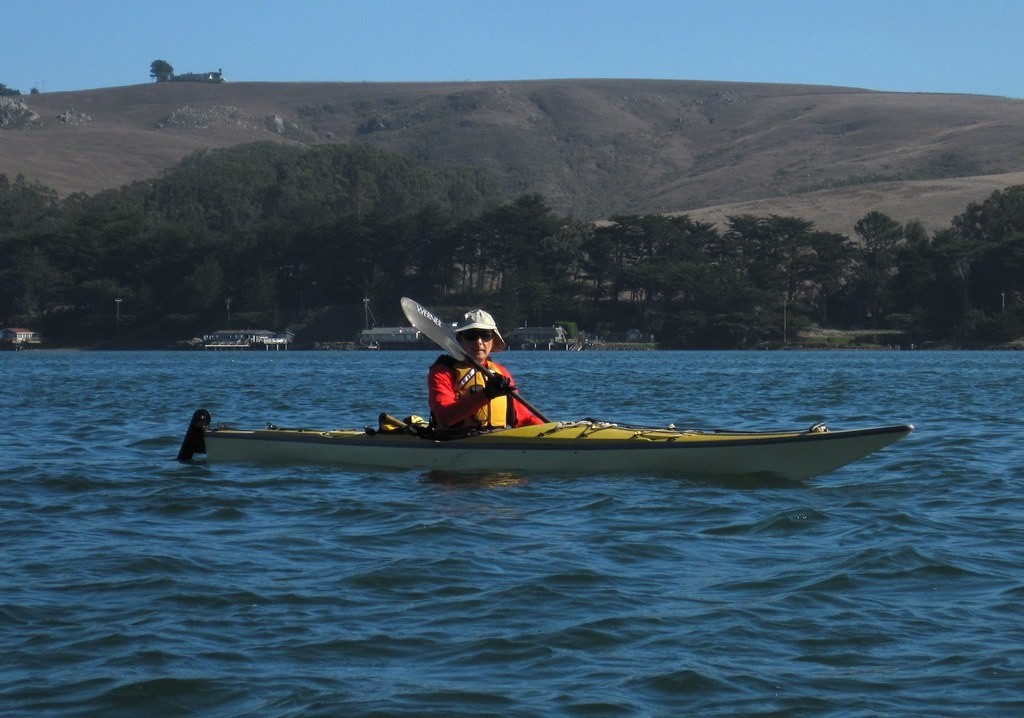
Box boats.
[178,408,914,487]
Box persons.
[428,309,545,436]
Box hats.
[453,309,505,353]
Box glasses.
[458,332,494,342]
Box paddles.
[400,295,552,423]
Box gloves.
[485,372,517,398]
